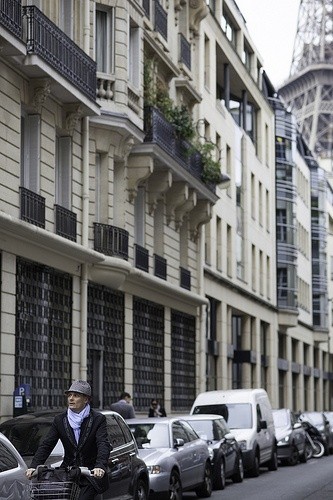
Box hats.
[64,380,91,396]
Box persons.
[148,398,166,417]
[111,392,136,419]
[24,379,109,500]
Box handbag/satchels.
[37,466,73,500]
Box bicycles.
[26,467,99,500]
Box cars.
[323,410,333,428]
[273,408,325,466]
[298,412,333,457]
[176,414,244,490]
[0,432,31,500]
[124,419,211,500]
[2,411,150,500]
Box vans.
[191,389,278,477]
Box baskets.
[30,481,74,500]
